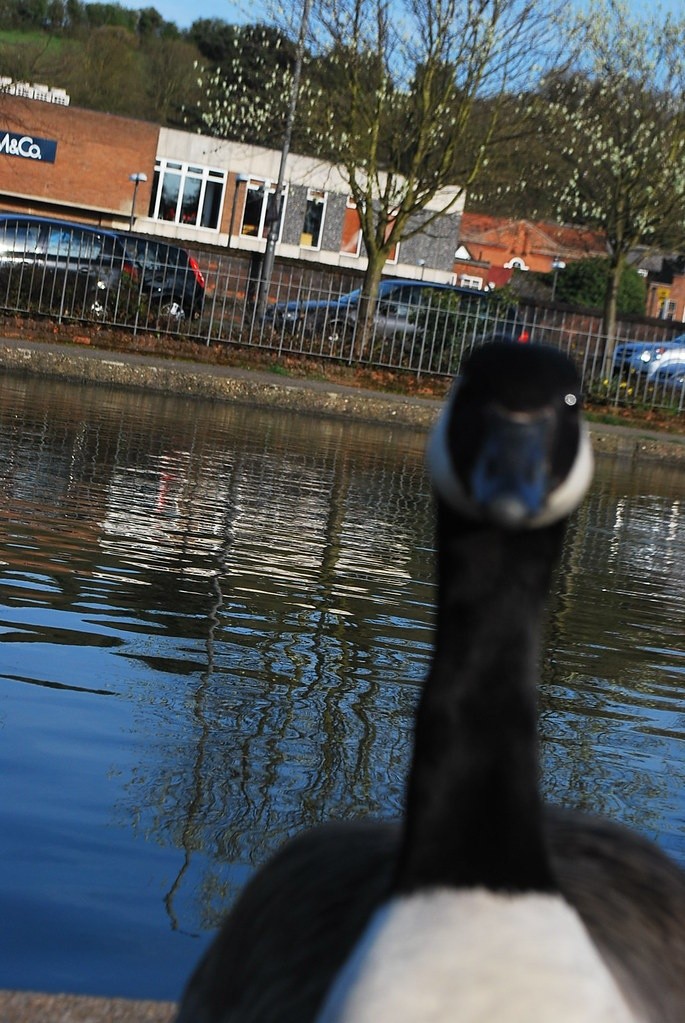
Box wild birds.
[172,331,684,1023]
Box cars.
[0,212,140,322]
[261,278,529,368]
[613,333,685,387]
[49,227,204,332]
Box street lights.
[551,261,566,300]
[129,173,148,230]
[419,258,426,281]
[228,174,249,247]
